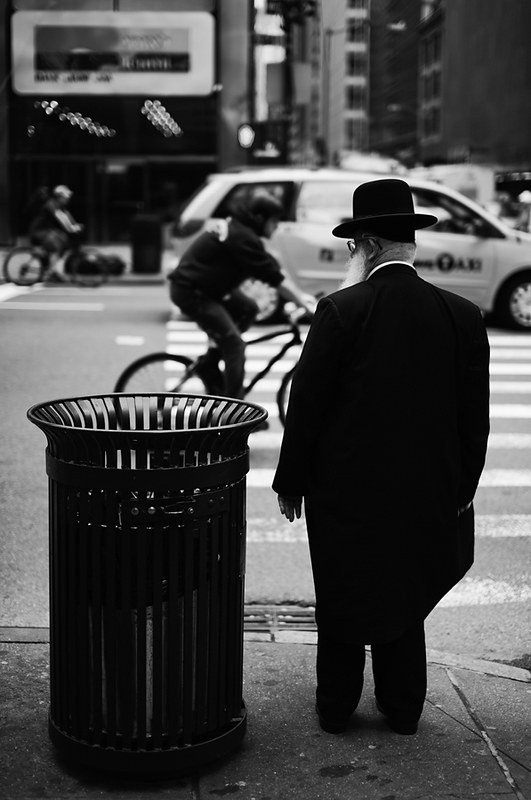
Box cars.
[162,164,531,332]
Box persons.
[272,178,490,736]
[28,184,82,281]
[513,191,531,234]
[166,193,319,424]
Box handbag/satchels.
[394,502,477,620]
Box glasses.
[347,238,382,253]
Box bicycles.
[115,292,323,428]
[3,227,109,287]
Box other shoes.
[379,698,419,735]
[51,268,69,282]
[315,695,348,733]
[193,354,221,385]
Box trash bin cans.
[23,391,271,788]
[131,212,165,274]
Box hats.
[332,179,438,239]
